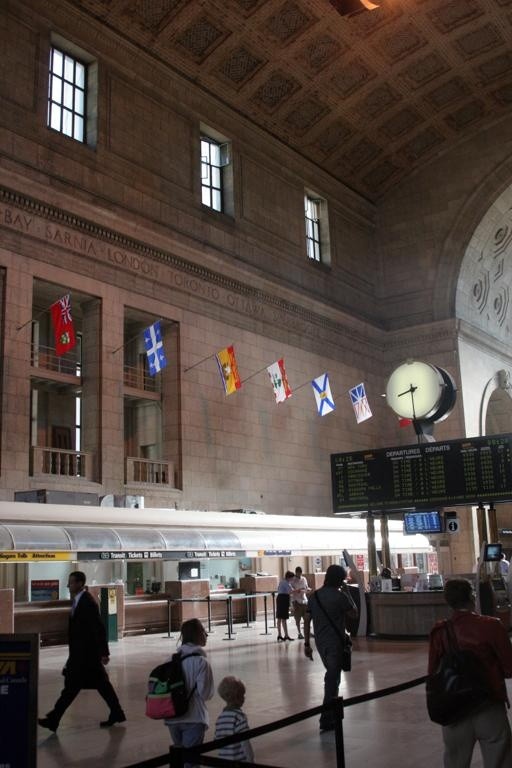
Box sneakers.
[298,633,304,639]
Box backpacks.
[145,649,201,720]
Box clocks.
[379,357,459,444]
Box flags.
[312,373,336,416]
[216,344,242,397]
[266,357,292,405]
[48,294,77,355]
[348,381,373,425]
[398,416,413,429]
[143,322,169,378]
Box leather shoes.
[37,716,59,732]
[100,711,126,726]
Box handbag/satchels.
[426,619,479,726]
[341,632,352,672]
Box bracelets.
[303,641,310,647]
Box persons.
[426,578,511,768]
[163,617,212,754]
[291,566,315,639]
[275,571,305,642]
[39,570,128,731]
[215,677,257,766]
[498,552,510,577]
[303,565,359,731]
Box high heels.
[277,635,294,641]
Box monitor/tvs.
[489,578,508,593]
[404,511,441,534]
[484,544,501,561]
